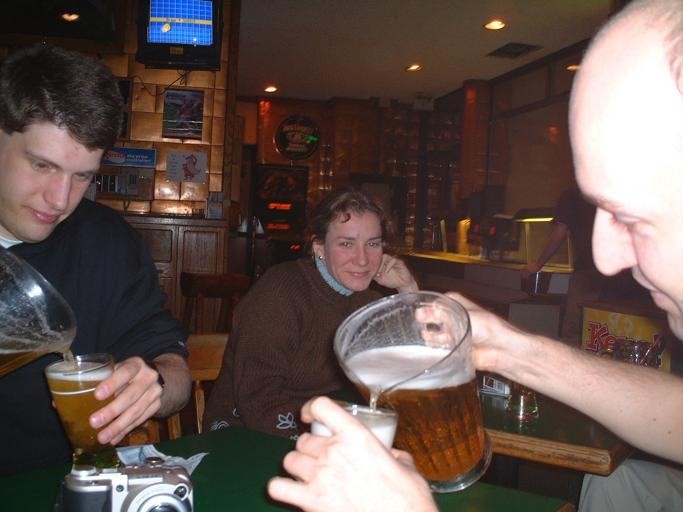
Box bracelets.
[148,359,167,388]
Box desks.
[2,425,577,511]
[328,382,631,504]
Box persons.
[203,185,418,439]
[519,180,627,342]
[0,42,192,476]
[268,0,681,512]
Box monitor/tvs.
[139,1,221,68]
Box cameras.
[62,456,194,512]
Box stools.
[177,268,251,334]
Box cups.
[43,351,116,452]
[333,287,494,495]
[313,399,400,453]
[0,243,77,378]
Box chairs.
[154,333,233,440]
